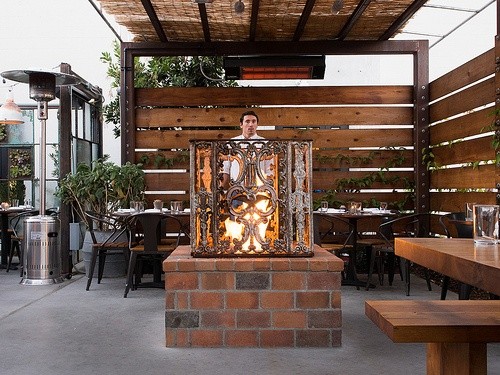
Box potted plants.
[54,154,144,278]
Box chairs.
[310,210,458,297]
[0,207,60,278]
[84,212,189,299]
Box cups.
[380,202,387,212]
[130,201,144,214]
[474,243,498,262]
[351,202,361,214]
[472,205,498,243]
[465,203,476,221]
[321,201,328,212]
[171,201,183,214]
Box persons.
[493,211,500,239]
[219,111,275,220]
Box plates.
[319,209,345,213]
[364,209,391,213]
[145,208,171,215]
[117,208,134,214]
[5,199,34,210]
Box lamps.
[0,82,25,125]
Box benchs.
[364,300,500,374]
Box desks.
[0,205,33,268]
[395,235,500,297]
[114,205,458,289]
